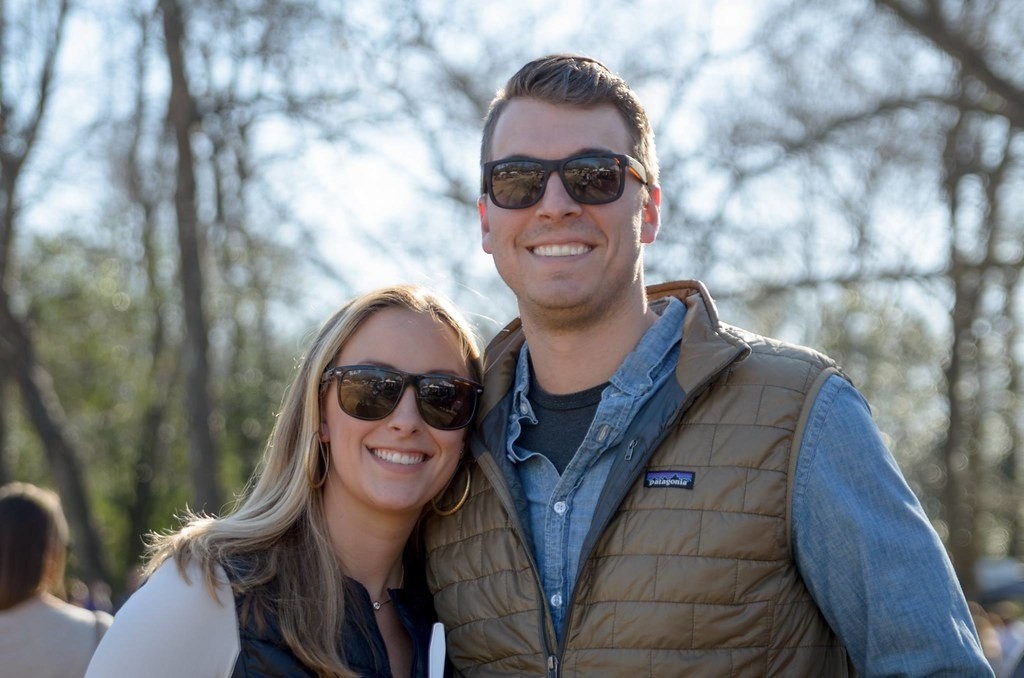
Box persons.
[405,56,995,678]
[82,288,485,678]
[0,480,115,678]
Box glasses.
[480,153,648,210]
[321,363,487,430]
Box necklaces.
[371,559,406,610]
